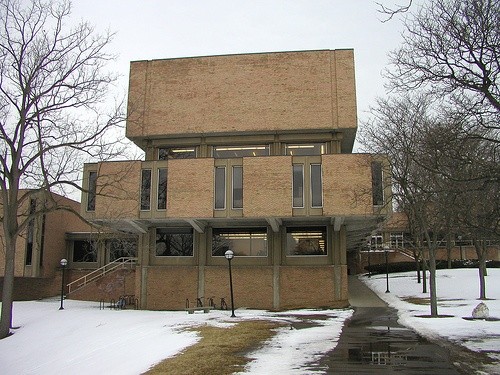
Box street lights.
[59,258,68,310]
[225,250,237,317]
[366,242,372,278]
[384,246,391,293]
